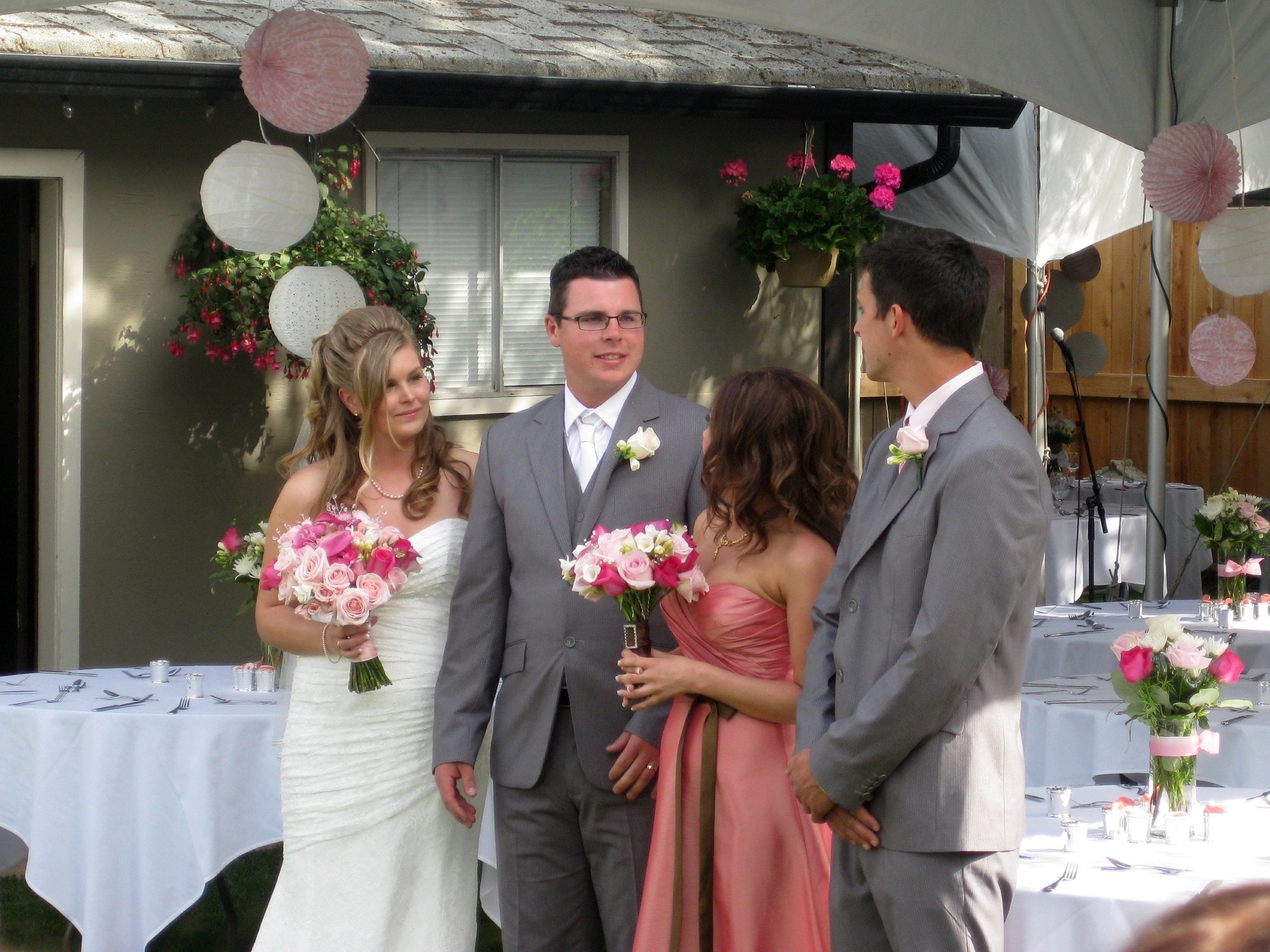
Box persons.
[251,305,501,952]
[432,241,712,952]
[613,364,864,952]
[788,227,1052,952]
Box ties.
[575,420,604,468]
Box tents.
[601,0,1270,607]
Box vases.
[1218,548,1245,619]
[1048,459,1069,500]
[776,247,839,288]
[1148,718,1198,839]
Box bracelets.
[322,622,341,664]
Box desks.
[1004,785,1270,952]
[0,665,501,952]
[1045,481,1214,604]
[1022,600,1270,788]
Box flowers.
[887,425,929,491]
[165,142,441,394]
[208,517,267,618]
[1047,404,1076,449]
[260,494,423,692]
[1191,486,1270,551]
[559,517,711,657]
[613,426,660,471]
[1110,614,1253,728]
[719,150,901,270]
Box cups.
[1045,785,1191,854]
[1257,681,1270,707]
[1199,592,1270,629]
[1128,599,1142,619]
[150,659,205,698]
[232,666,276,694]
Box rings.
[647,763,659,774]
[337,640,341,650]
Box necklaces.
[713,508,755,561]
[365,456,427,500]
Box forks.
[9,690,72,707]
[1031,599,1168,631]
[167,697,192,714]
[1021,674,1112,695]
[122,670,151,679]
[91,693,154,711]
[1119,784,1152,795]
[0,675,29,686]
[1240,667,1250,674]
[1043,863,1079,892]
[58,685,72,692]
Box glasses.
[554,312,647,331]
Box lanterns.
[200,7,368,358]
[1138,119,1270,386]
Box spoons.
[72,680,86,693]
[1077,602,1171,630]
[210,695,277,704]
[1108,857,1194,874]
[103,689,159,701]
[139,667,181,676]
[1239,673,1267,681]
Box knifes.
[1021,683,1128,704]
[1044,603,1108,638]
[39,670,98,676]
[0,690,38,694]
[1221,716,1246,726]
